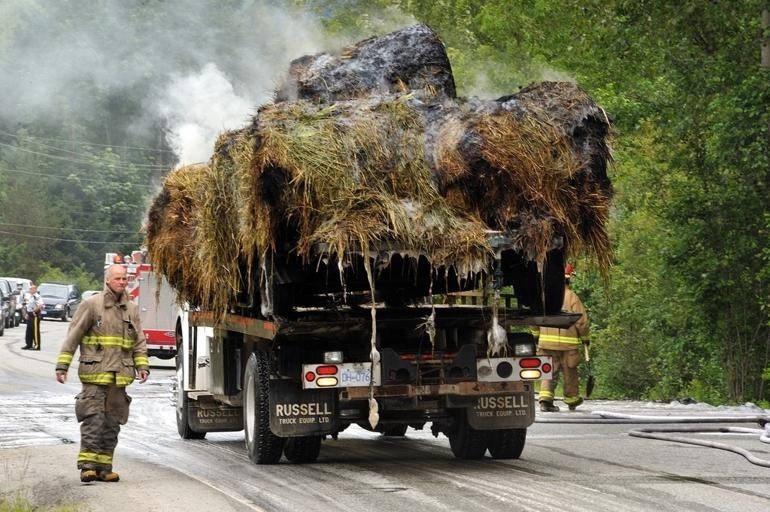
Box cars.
[82,290,102,299]
[0,276,35,335]
[37,281,82,321]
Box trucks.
[175,230,582,463]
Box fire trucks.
[105,253,180,359]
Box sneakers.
[80,469,120,482]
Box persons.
[55,264,153,484]
[535,264,593,411]
[21,285,45,352]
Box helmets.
[565,263,574,274]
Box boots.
[569,397,583,410]
[540,402,559,412]
[22,345,36,349]
